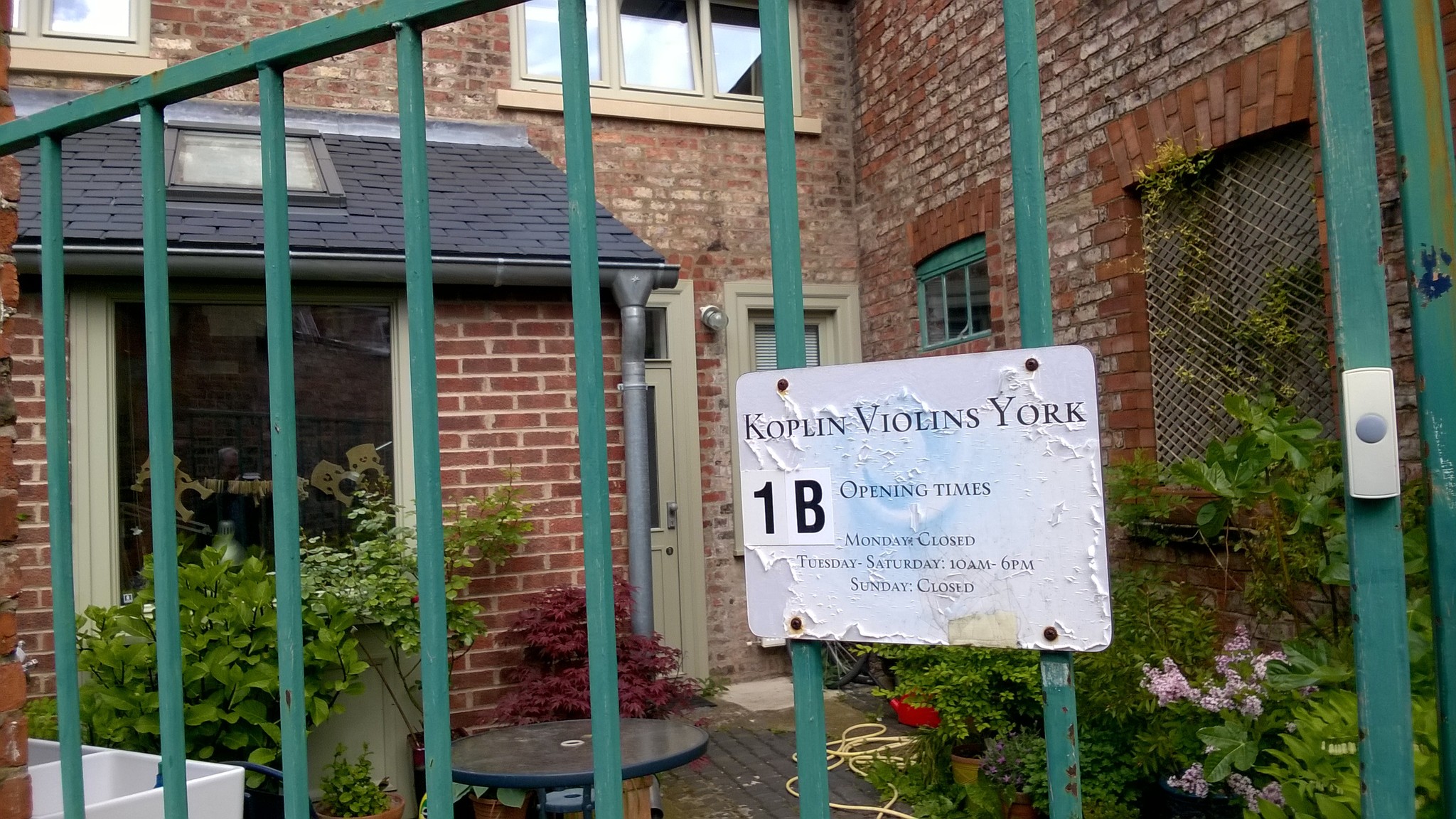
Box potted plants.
[313,743,405,819]
[1151,466,1221,524]
[951,702,982,785]
[304,457,534,819]
[1230,470,1289,529]
[452,782,530,819]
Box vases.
[1002,792,1037,819]
[1154,769,1238,818]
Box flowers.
[979,732,1024,787]
[1140,659,1246,797]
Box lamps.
[699,305,728,331]
[120,503,247,567]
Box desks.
[452,718,710,819]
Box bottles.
[154,762,163,788]
